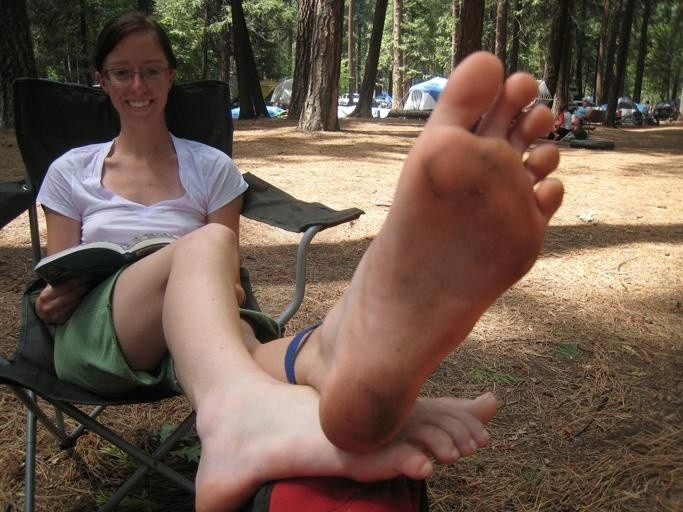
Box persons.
[550,96,651,142]
[28,7,567,509]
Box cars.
[374,97,388,108]
[337,92,359,106]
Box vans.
[573,99,678,123]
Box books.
[33,236,183,289]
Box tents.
[270,80,294,106]
[404,75,447,112]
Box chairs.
[1,75,369,512]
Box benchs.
[547,110,662,144]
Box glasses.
[99,66,172,81]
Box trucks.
[567,86,579,112]
